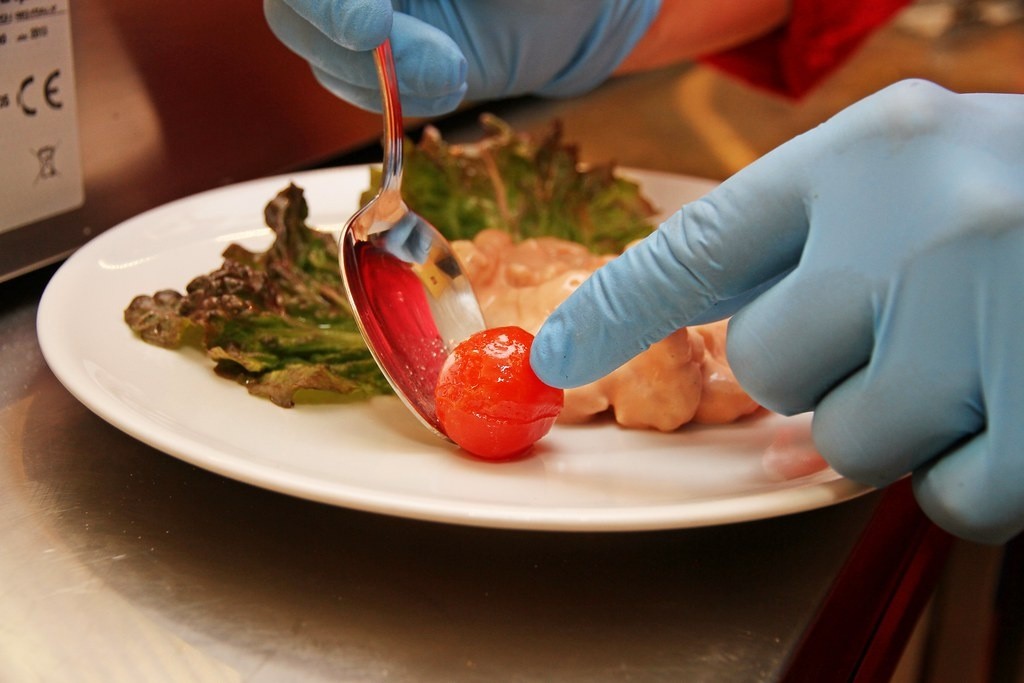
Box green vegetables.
[122,110,693,409]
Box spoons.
[337,39,487,448]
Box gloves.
[264,0,661,118]
[529,78,1024,549]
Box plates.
[36,162,915,534]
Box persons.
[263,0,1024,549]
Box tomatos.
[433,325,564,461]
